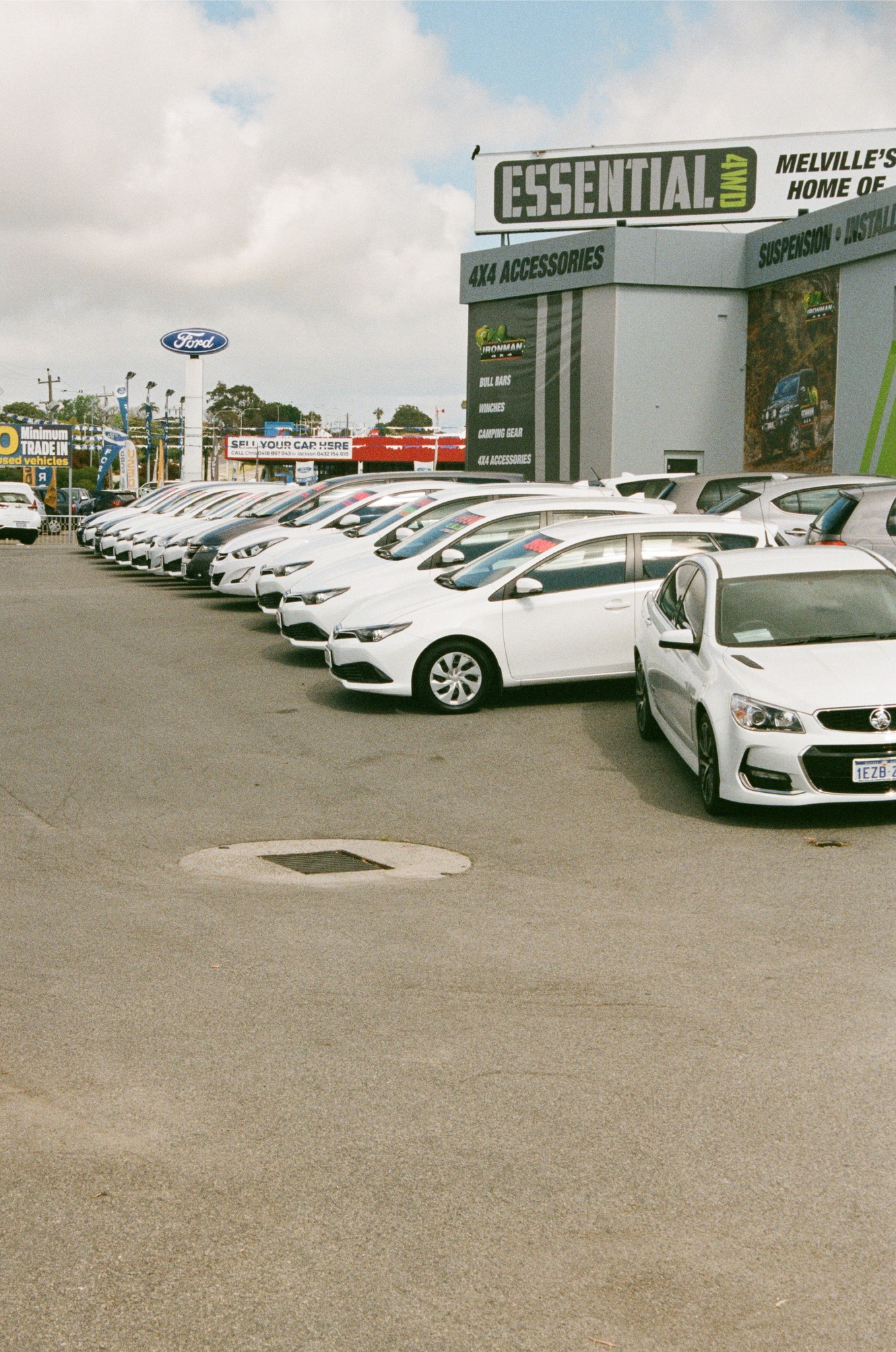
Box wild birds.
[471,145,480,160]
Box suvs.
[29,485,76,535]
[88,489,137,512]
[61,486,95,516]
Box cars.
[0,460,896,719]
[322,511,793,714]
[630,542,896,821]
[805,483,896,568]
[702,474,896,547]
